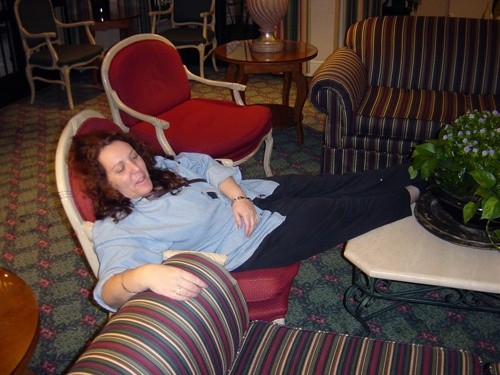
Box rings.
[176,287,181,295]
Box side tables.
[214,38,318,144]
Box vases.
[414,176,500,250]
[246,0,289,54]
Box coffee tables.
[344,190,500,359]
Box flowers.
[408,110,500,249]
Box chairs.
[148,0,218,78]
[13,0,106,110]
[55,109,301,321]
[101,34,276,182]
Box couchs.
[311,15,500,179]
[64,254,500,375]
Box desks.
[0,266,41,375]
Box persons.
[71,127,426,313]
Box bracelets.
[231,196,251,206]
[121,269,140,294]
